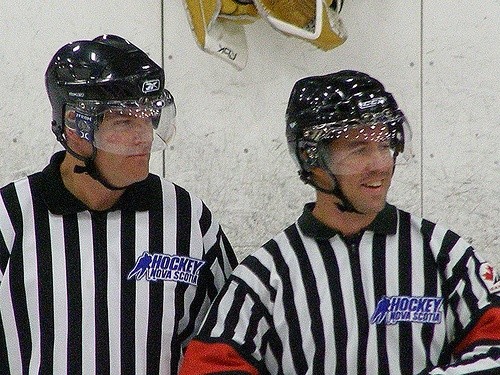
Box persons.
[0,35,239,375]
[178,71,500,375]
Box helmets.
[286,70,405,172]
[45,34,174,137]
